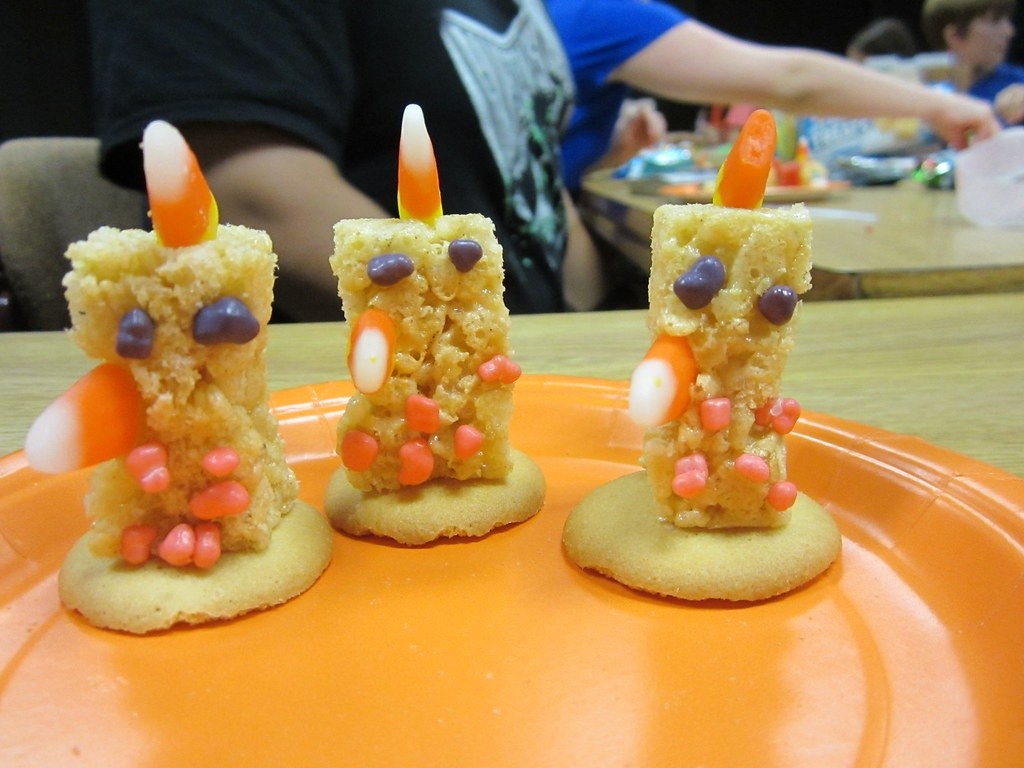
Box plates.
[0,373,1024,768]
[657,179,854,201]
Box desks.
[575,141,1024,302]
[0,289,1024,768]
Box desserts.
[325,104,544,544]
[565,108,839,601]
[27,121,331,633]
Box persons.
[888,1,1023,132]
[99,0,1002,313]
